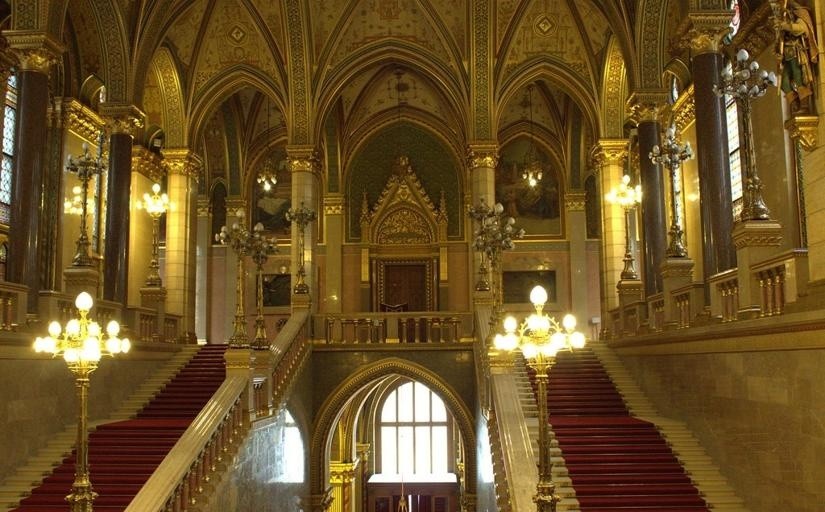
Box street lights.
[137,183,176,286]
[215,200,318,351]
[32,291,132,512]
[494,285,585,512]
[468,199,525,347]
[712,49,776,222]
[605,175,643,279]
[64,141,108,268]
[649,128,695,258]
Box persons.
[768,0,820,118]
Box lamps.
[522,85,543,187]
[256,96,278,193]
